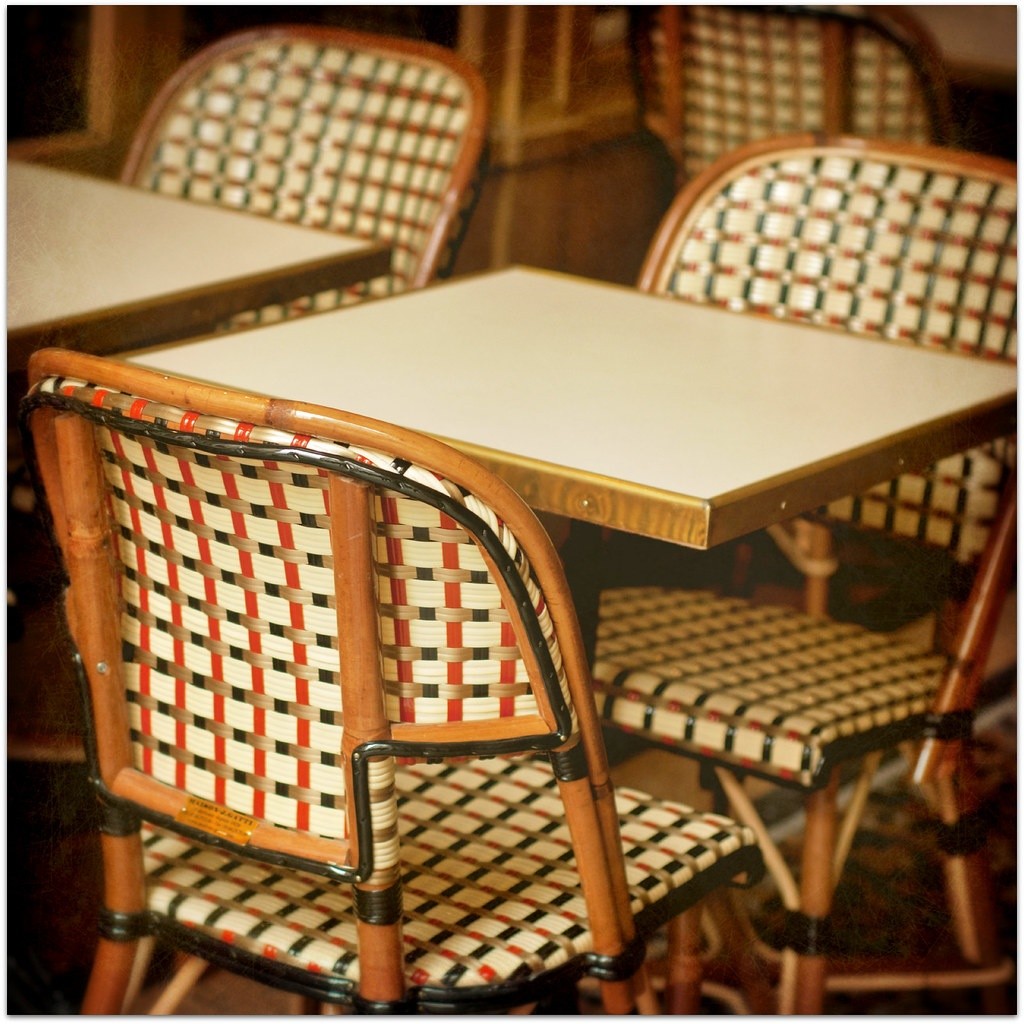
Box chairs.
[117,26,488,334]
[578,133,1017,1015]
[642,7,961,201]
[18,346,780,1017]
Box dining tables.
[109,260,1016,1015]
[919,8,1016,92]
[8,155,396,368]
